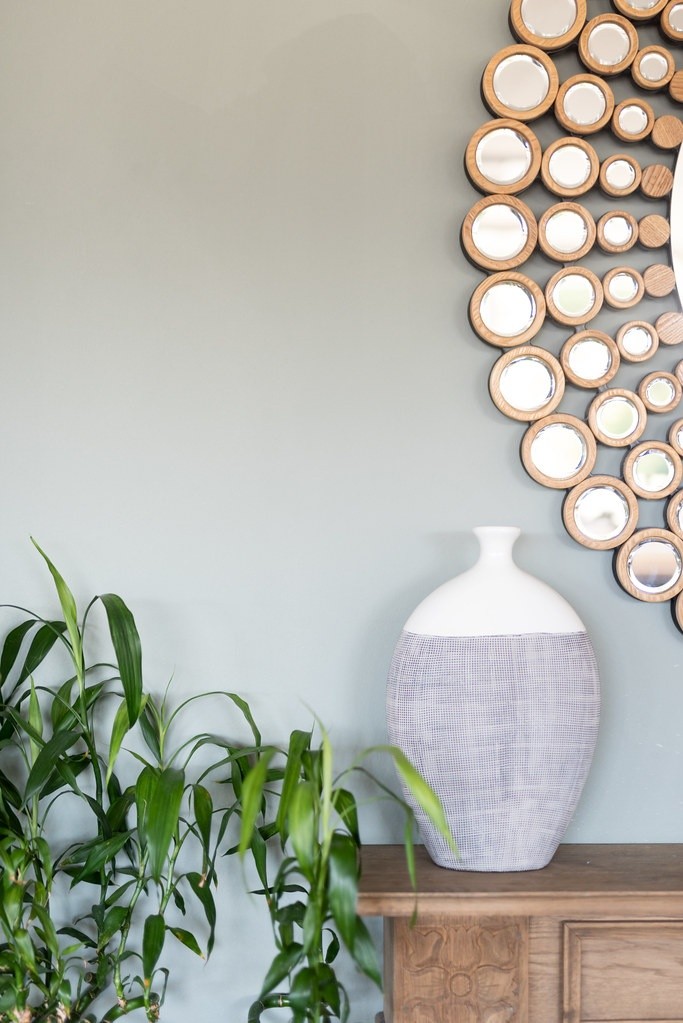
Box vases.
[387,524,599,870]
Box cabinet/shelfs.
[353,842,683,1023]
